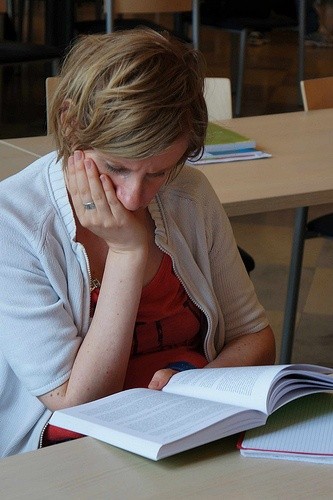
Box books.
[48,364,333,465]
[185,121,272,165]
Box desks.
[1,436,333,500]
[1,107,333,364]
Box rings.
[83,201,96,210]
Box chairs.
[298,76,333,114]
[105,0,199,91]
[193,75,232,120]
[188,0,318,115]
[0,39,61,75]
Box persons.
[0,26,276,461]
